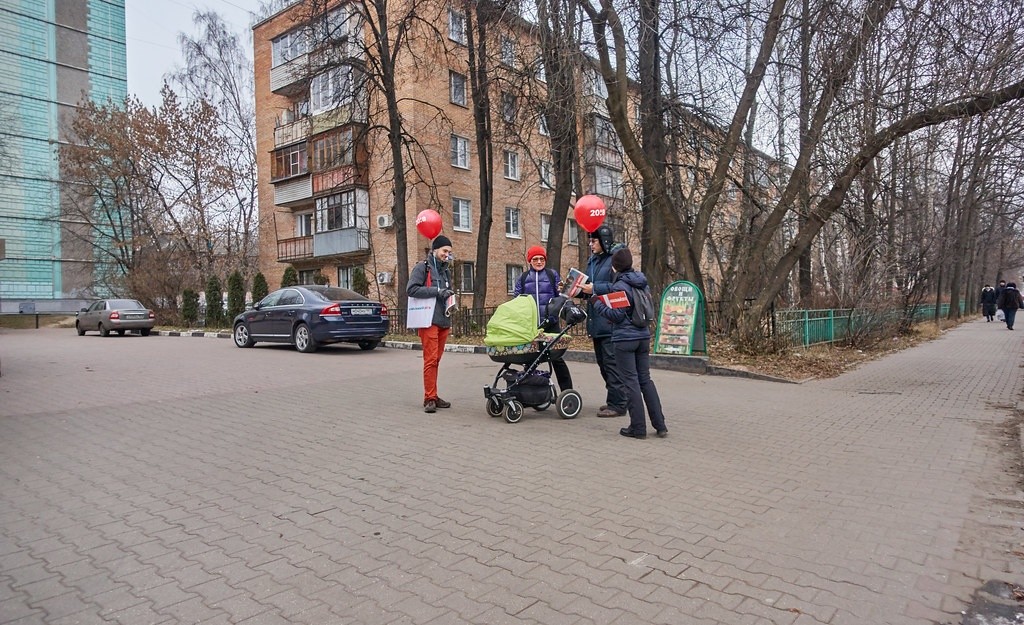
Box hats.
[432,235,452,250]
[612,247,632,271]
[527,246,547,263]
[586,226,613,254]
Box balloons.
[415,209,442,239]
[574,195,606,233]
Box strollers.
[483,294,588,424]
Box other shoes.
[599,405,627,416]
[597,408,625,417]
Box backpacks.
[613,280,654,327]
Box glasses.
[532,257,545,263]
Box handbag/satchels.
[406,260,436,328]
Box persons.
[513,245,573,392]
[406,235,454,414]
[590,248,668,439]
[979,285,996,322]
[557,226,628,417]
[993,280,1024,330]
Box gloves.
[590,294,597,303]
[439,288,454,298]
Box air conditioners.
[376,272,394,286]
[377,214,394,231]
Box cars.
[233,286,391,353]
[76,299,157,337]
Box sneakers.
[425,401,436,412]
[423,398,450,408]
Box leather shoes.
[620,427,646,438]
[658,428,667,436]
